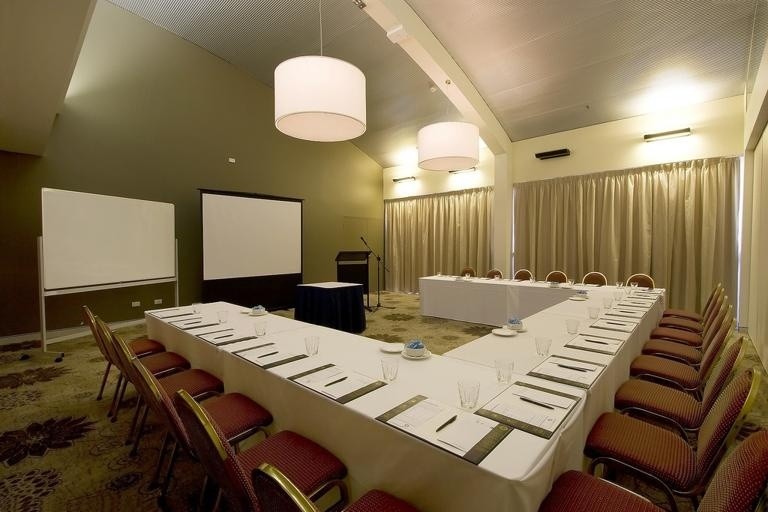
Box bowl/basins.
[405,347,425,356]
[508,321,522,330]
[252,307,265,313]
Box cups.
[614,291,623,301]
[588,307,600,319]
[255,320,264,335]
[530,277,533,284]
[381,357,399,381]
[304,336,319,356]
[495,360,514,384]
[616,282,623,288]
[624,289,631,294]
[630,282,638,288]
[437,272,441,276]
[192,303,200,313]
[494,275,500,281]
[218,311,227,323]
[535,336,552,356]
[568,279,574,286]
[567,320,579,333]
[603,299,612,309]
[457,377,480,408]
[465,273,470,278]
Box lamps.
[535,149,571,160]
[392,176,415,185]
[644,127,690,141]
[417,80,480,173]
[449,168,474,174]
[273,0,367,142]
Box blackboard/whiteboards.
[41,188,177,292]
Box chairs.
[80,268,768,512]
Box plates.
[492,328,516,337]
[504,324,525,332]
[401,351,431,359]
[639,287,649,290]
[569,295,588,301]
[240,309,250,313]
[381,343,404,352]
[250,311,267,316]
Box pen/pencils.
[258,352,278,357]
[521,397,555,410]
[637,294,649,297]
[214,334,233,339]
[184,320,200,325]
[632,301,645,305]
[621,311,635,314]
[325,377,347,387]
[436,416,457,433]
[558,364,586,373]
[586,338,608,344]
[608,321,627,326]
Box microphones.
[360,236,368,245]
